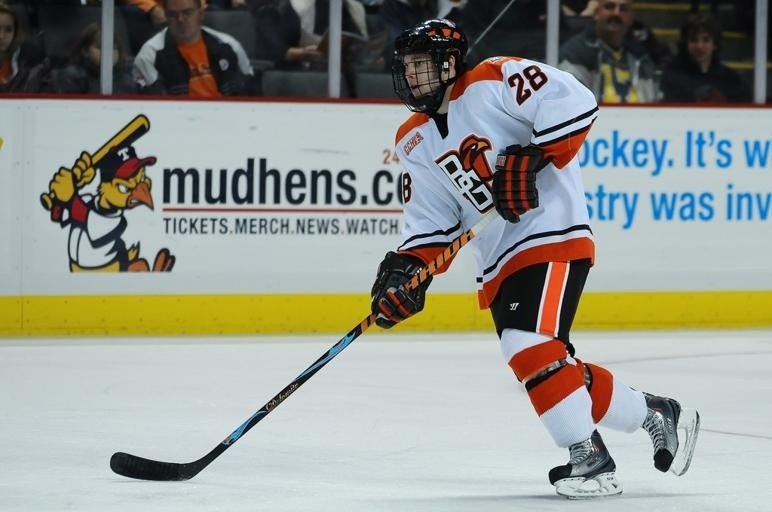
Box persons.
[368,15,684,488]
[0,0,772,108]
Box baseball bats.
[40,114,151,210]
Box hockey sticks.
[109,157,554,481]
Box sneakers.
[549,428,615,485]
[641,391,681,472]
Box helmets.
[390,18,468,113]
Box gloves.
[370,251,432,329]
[492,144,545,224]
